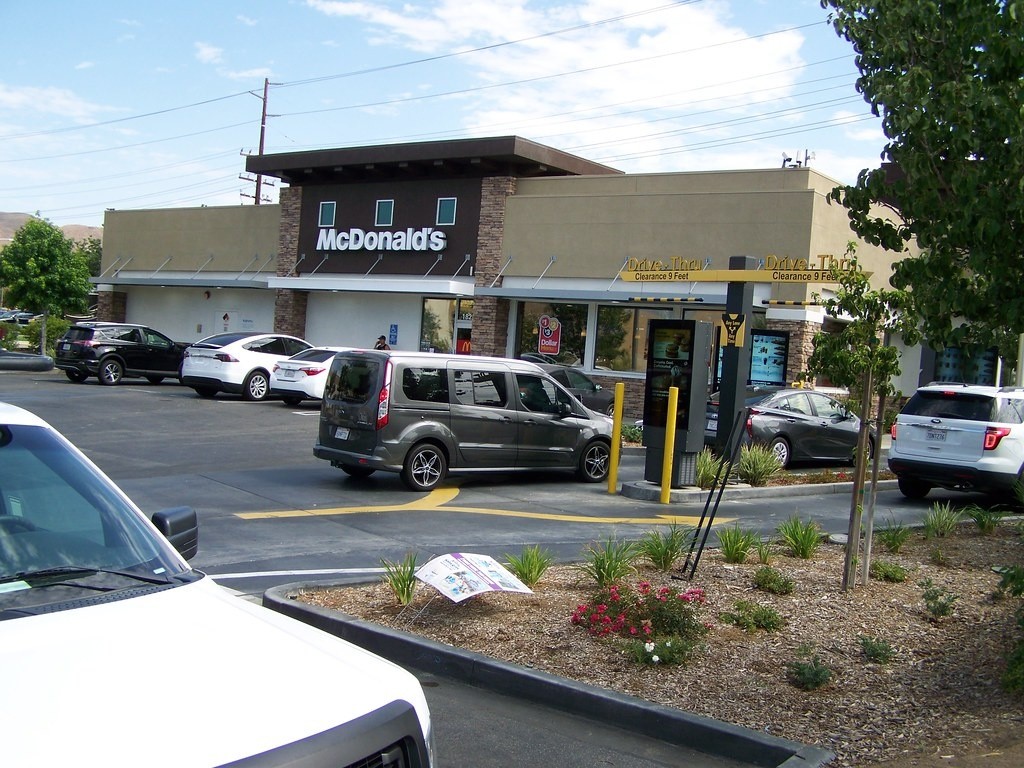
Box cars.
[519,362,616,420]
[519,352,582,367]
[181,331,317,402]
[0,308,44,324]
[270,346,358,405]
[0,402,435,768]
[704,384,878,471]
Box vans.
[312,348,624,492]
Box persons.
[374,335,390,350]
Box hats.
[377,335,386,340]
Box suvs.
[887,381,1024,514]
[53,320,196,386]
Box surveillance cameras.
[796,150,804,163]
[782,152,792,162]
